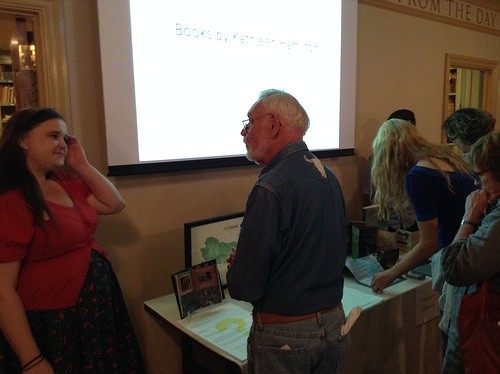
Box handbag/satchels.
[456,287,500,374]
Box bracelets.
[21,354,45,372]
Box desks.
[143,252,446,374]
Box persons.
[0,106,126,374]
[370,109,500,374]
[226,88,352,374]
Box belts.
[252,309,330,324]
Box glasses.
[473,169,495,183]
[242,112,283,134]
[446,134,457,144]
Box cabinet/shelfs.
[0,50,17,136]
[447,68,462,118]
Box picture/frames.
[183,210,245,290]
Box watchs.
[460,220,476,227]
